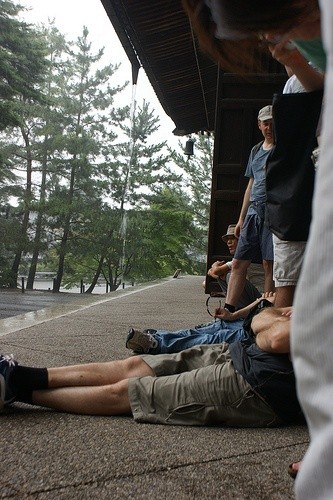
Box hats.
[221,224,236,242]
[258,105,272,121]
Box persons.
[0,305,309,427]
[190,0,332,499]
[270,60,326,307]
[125,291,277,355]
[223,105,275,313]
[207,224,276,311]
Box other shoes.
[125,329,151,352]
